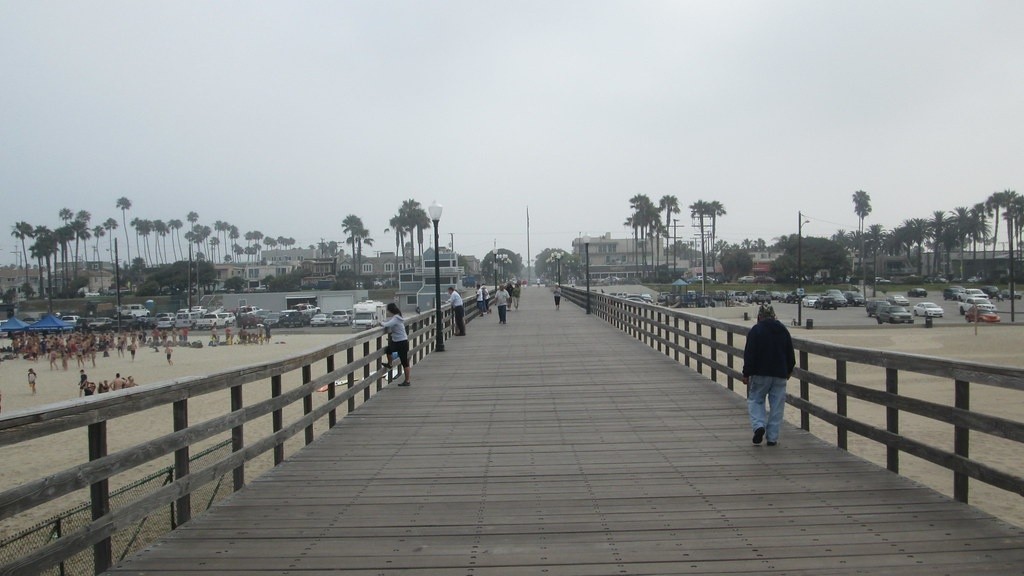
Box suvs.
[980,285,1002,299]
[331,309,354,327]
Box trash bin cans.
[744,312,749,320]
[806,318,813,329]
[926,316,932,328]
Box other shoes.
[398,380,410,386]
[455,332,465,336]
[752,427,765,444]
[767,440,778,446]
[382,362,393,369]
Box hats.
[758,304,775,317]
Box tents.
[0,316,30,332]
[24,314,74,334]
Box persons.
[445,286,466,336]
[27,368,37,393]
[742,304,796,446]
[553,285,561,309]
[537,277,540,287]
[475,283,490,317]
[494,284,510,324]
[77,370,138,397]
[379,303,410,386]
[523,279,526,288]
[8,327,189,369]
[505,280,521,311]
[211,325,271,343]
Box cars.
[609,275,868,311]
[866,300,892,317]
[873,274,984,285]
[59,304,330,330]
[943,285,965,301]
[960,296,993,315]
[957,288,989,303]
[876,305,915,324]
[907,287,928,298]
[965,305,1001,323]
[913,301,945,318]
[1001,289,1022,300]
[886,294,910,307]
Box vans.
[350,300,387,331]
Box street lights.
[583,233,592,314]
[493,246,498,294]
[105,248,122,334]
[184,257,192,312]
[428,200,445,352]
[798,220,811,326]
[556,253,562,288]
